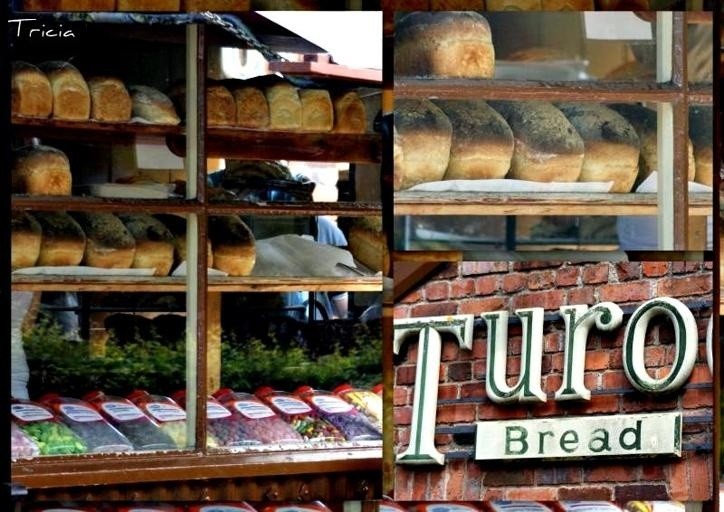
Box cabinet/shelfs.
[1,11,383,512]
[393,10,715,250]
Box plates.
[493,57,592,82]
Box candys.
[11,390,383,457]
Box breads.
[395,10,496,82]
[392,98,713,190]
[11,59,182,124]
[16,145,72,196]
[205,84,365,135]
[10,208,258,275]
